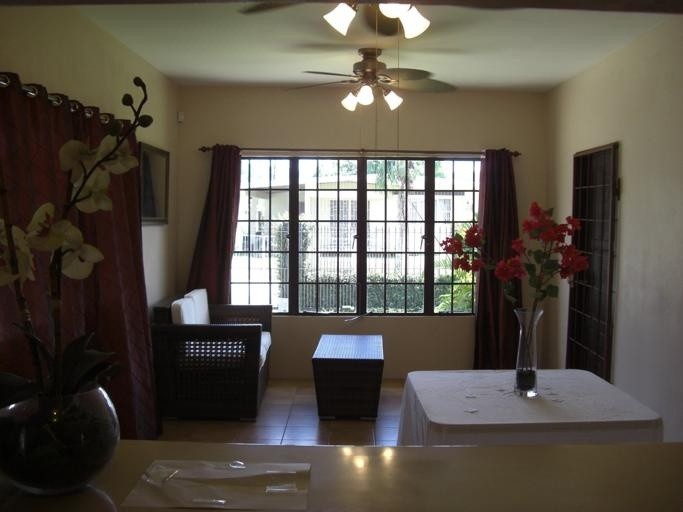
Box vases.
[513,307,544,397]
[0,384,122,498]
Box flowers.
[440,201,589,371]
[0,75,154,391]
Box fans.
[285,46,457,93]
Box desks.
[407,370,663,445]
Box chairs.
[150,286,272,423]
